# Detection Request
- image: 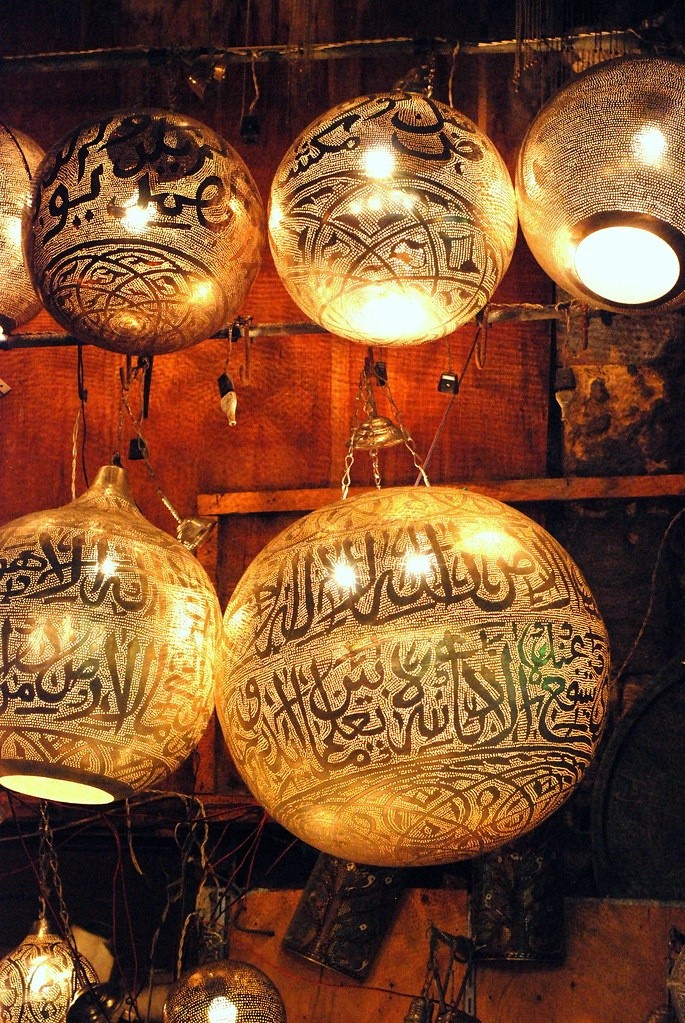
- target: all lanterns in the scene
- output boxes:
[0,920,289,1023]
[1,55,684,862]
